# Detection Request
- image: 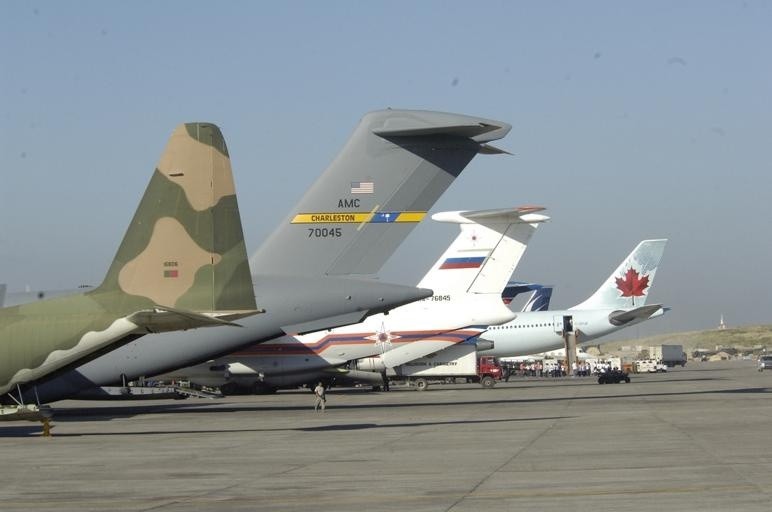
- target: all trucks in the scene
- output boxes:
[649,344,687,366]
[384,344,504,393]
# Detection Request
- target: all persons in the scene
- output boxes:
[372,369,382,392]
[381,367,391,392]
[313,380,328,414]
[508,360,612,378]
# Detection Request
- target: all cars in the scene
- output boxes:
[757,355,772,373]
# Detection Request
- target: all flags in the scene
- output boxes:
[437,249,492,271]
[350,181,375,194]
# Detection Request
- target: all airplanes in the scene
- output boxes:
[128,204,670,395]
[0,108,518,419]
